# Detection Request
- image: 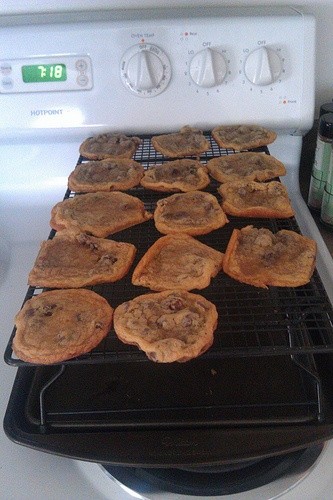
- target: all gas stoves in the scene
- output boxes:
[1,8,332,499]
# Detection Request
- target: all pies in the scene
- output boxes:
[221,224,318,290]
[153,191,229,236]
[140,159,211,192]
[28,232,136,287]
[151,124,211,157]
[211,124,277,151]
[68,157,144,191]
[217,180,295,218]
[206,152,287,183]
[10,289,114,366]
[79,132,144,158]
[131,233,225,291]
[114,289,218,363]
[49,191,154,239]
